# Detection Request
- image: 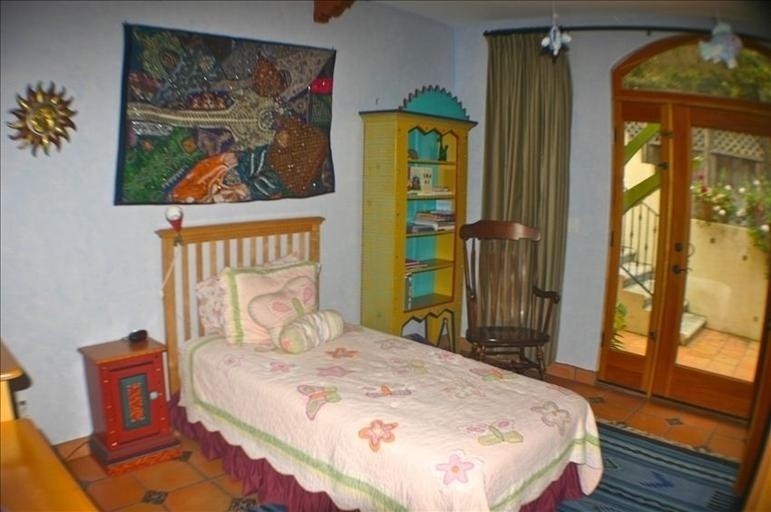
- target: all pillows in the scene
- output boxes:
[192,251,347,353]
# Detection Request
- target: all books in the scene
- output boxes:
[406,258,428,269]
[405,275,413,309]
[407,209,456,233]
[408,166,452,196]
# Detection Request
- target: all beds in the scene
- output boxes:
[160,213,591,511]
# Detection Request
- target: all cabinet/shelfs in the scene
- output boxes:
[358,108,479,357]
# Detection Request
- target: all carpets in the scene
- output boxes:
[552,413,744,512]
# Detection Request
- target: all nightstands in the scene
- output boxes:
[74,340,183,478]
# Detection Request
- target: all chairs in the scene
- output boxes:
[456,218,561,381]
